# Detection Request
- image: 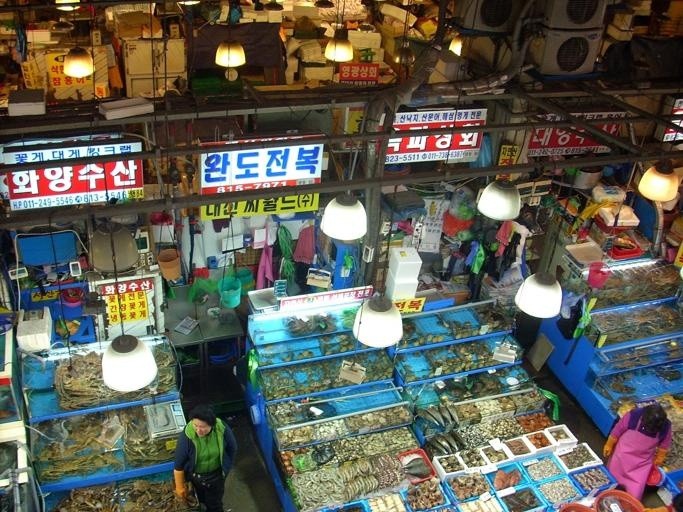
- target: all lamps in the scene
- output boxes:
[448,0,473,56]
[637,110,683,201]
[476,102,591,318]
[62,1,94,78]
[214,0,247,69]
[393,0,416,66]
[317,102,405,351]
[90,157,158,394]
[323,0,356,64]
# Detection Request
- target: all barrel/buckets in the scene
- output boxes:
[218,278,241,309]
[237,269,254,296]
[158,249,181,280]
[586,261,611,288]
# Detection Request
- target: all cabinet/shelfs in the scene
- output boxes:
[0,328,193,511]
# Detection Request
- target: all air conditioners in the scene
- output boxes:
[464,0,610,82]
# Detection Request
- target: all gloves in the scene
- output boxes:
[602,435,618,458]
[653,447,668,465]
[172,468,186,498]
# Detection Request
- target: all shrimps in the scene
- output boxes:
[290,453,406,512]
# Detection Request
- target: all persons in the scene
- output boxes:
[170,404,237,511]
[602,404,672,502]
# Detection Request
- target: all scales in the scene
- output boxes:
[143,400,186,439]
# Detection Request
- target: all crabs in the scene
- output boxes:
[37,346,198,512]
[586,303,683,370]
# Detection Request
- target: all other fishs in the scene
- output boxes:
[415,400,471,457]
[401,457,433,478]
